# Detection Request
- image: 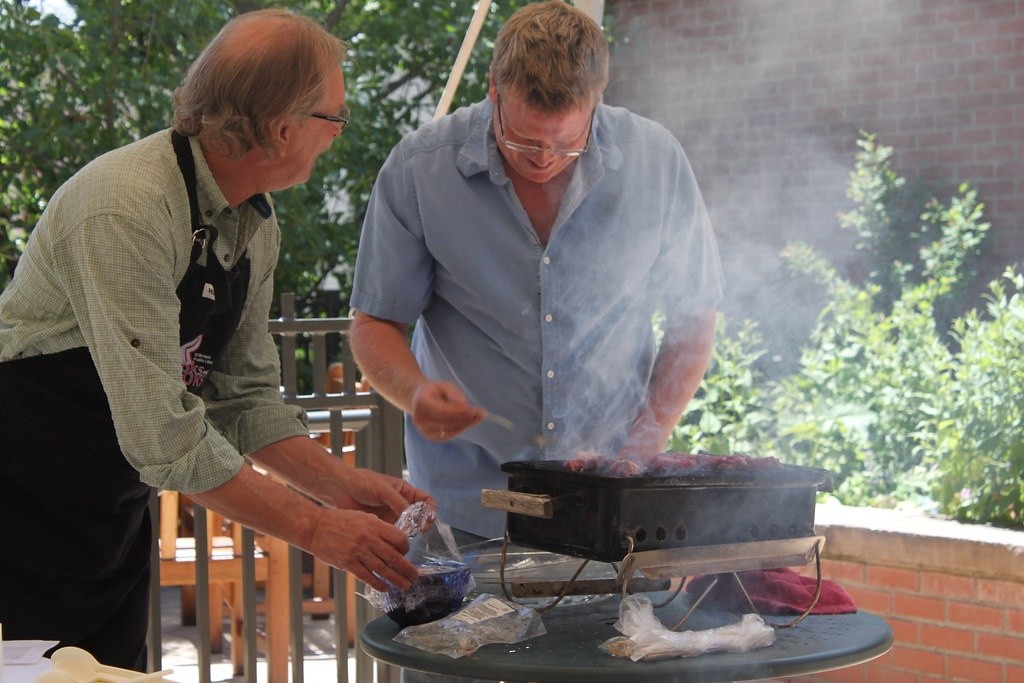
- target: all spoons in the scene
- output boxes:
[35,646,178,683]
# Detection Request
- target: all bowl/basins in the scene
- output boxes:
[377,561,471,629]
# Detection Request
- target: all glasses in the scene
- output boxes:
[497,92,596,156]
[311,105,350,129]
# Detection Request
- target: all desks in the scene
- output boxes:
[361,591,896,683]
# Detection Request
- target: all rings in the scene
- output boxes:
[438,425,445,437]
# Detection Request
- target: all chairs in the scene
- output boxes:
[160,484,290,683]
[178,363,371,655]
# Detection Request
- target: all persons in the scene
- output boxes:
[349,1,725,564]
[0,10,437,671]
